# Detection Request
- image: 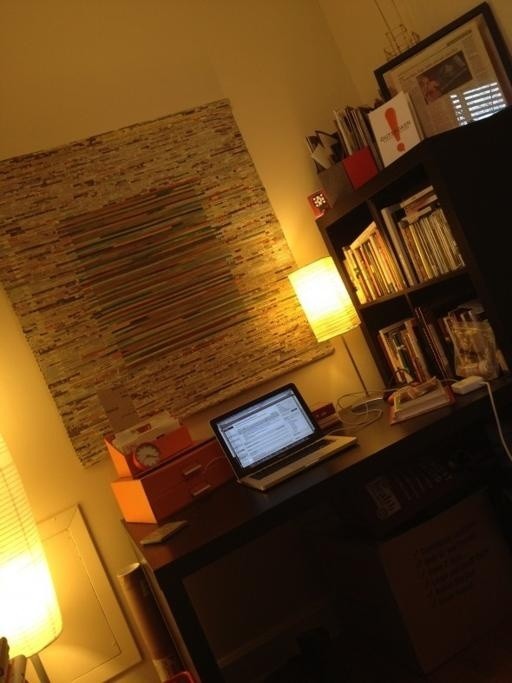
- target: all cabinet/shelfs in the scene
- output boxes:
[314,105,511,393]
[109,434,235,523]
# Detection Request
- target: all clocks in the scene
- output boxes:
[129,439,162,470]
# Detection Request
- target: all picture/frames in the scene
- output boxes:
[371,0,512,135]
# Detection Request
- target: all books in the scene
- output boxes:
[390,377,455,424]
[341,221,405,305]
[377,317,437,385]
[381,185,468,287]
[304,93,428,174]
[416,298,503,380]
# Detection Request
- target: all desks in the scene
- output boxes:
[117,378,511,683]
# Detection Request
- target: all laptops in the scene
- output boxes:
[209,382,358,491]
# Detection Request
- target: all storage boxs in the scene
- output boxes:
[340,145,381,187]
[377,493,511,683]
[316,161,355,208]
[425,608,509,683]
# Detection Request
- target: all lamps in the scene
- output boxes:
[287,255,384,409]
[1,434,67,682]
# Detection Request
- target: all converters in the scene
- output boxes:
[450,376,485,395]
